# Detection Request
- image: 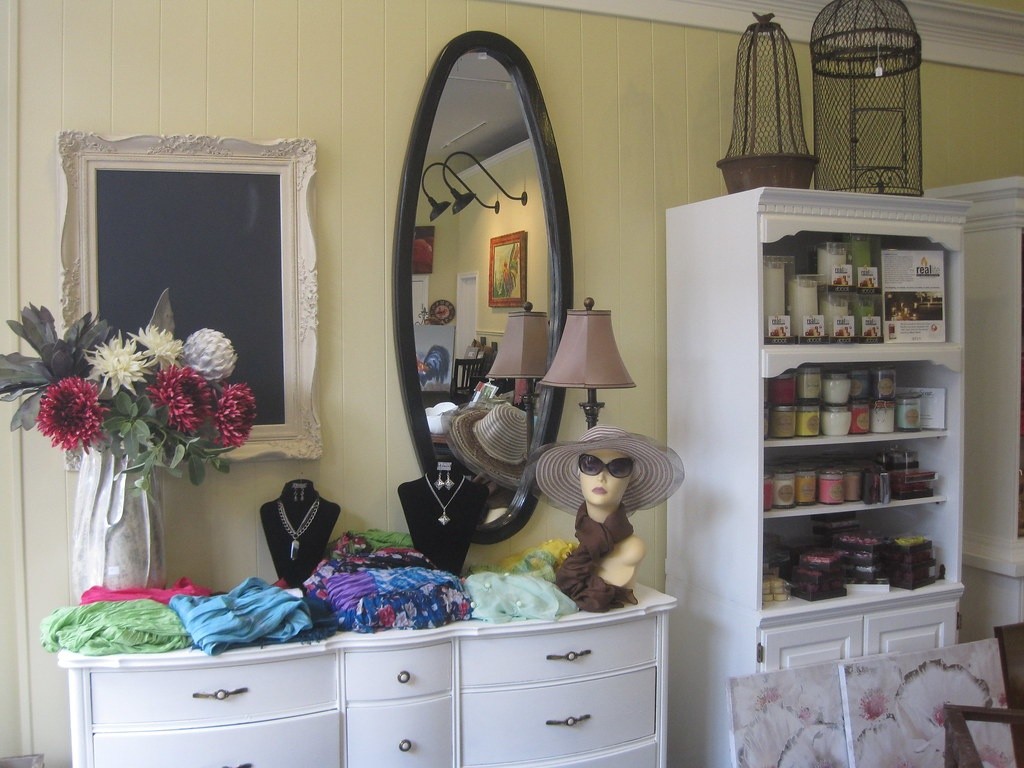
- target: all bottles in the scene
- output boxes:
[763,360,920,510]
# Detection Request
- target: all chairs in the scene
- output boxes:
[430,357,484,445]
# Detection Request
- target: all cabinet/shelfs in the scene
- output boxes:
[57,580,678,768]
[664,186,972,768]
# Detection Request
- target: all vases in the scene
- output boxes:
[72,447,169,602]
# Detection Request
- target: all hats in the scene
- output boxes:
[440,400,527,492]
[523,424,684,518]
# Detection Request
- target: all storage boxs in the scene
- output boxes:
[862,445,938,505]
[763,234,884,345]
[763,512,937,601]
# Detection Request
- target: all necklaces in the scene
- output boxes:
[425,473,466,525]
[277,494,319,562]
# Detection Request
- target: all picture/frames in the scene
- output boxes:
[52,128,327,465]
[488,230,528,308]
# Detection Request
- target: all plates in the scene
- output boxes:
[429,299,455,325]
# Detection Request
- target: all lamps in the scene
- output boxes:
[421,151,527,223]
[537,296,637,430]
[485,301,550,453]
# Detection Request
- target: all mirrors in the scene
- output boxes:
[391,29,578,548]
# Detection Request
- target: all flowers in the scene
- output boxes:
[0,300,258,507]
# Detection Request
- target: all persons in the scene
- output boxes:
[523,425,684,612]
[439,402,528,524]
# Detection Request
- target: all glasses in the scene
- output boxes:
[578,454,633,479]
